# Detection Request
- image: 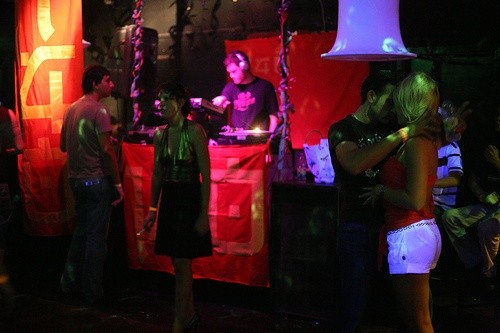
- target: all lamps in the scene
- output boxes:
[320,0,417,61]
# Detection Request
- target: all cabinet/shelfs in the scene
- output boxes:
[271,179,345,333]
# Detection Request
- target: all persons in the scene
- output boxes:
[60,65,125,311]
[190,51,278,143]
[134,80,212,333]
[326,72,442,333]
[431,105,500,292]
[358,72,443,333]
[0,100,30,222]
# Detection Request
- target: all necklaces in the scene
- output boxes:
[353,113,359,121]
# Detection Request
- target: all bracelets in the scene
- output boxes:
[148,206,157,212]
[113,183,122,188]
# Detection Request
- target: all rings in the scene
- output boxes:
[367,198,371,201]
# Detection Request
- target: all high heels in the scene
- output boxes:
[185,311,201,333]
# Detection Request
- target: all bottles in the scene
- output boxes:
[441,94,461,142]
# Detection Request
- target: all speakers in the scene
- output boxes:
[268,181,348,319]
[107,25,159,99]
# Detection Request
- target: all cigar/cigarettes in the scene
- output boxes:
[135,229,145,235]
